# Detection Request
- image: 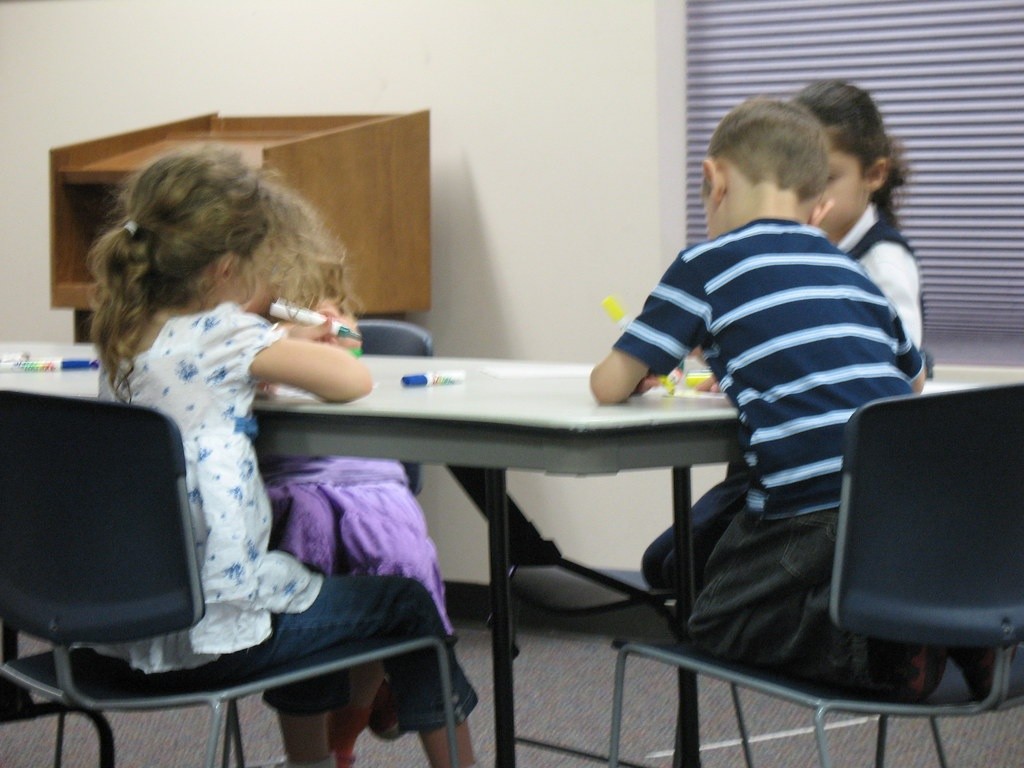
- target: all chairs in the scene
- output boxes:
[0,388,461,768]
[605,381,1024,768]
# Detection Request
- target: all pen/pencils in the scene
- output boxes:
[0,360,90,371]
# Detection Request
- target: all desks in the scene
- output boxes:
[0,344,739,768]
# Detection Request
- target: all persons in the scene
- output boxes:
[84,142,479,768]
[590,76,996,702]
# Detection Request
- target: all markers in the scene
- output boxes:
[601,296,675,394]
[401,375,459,388]
[269,298,369,344]
